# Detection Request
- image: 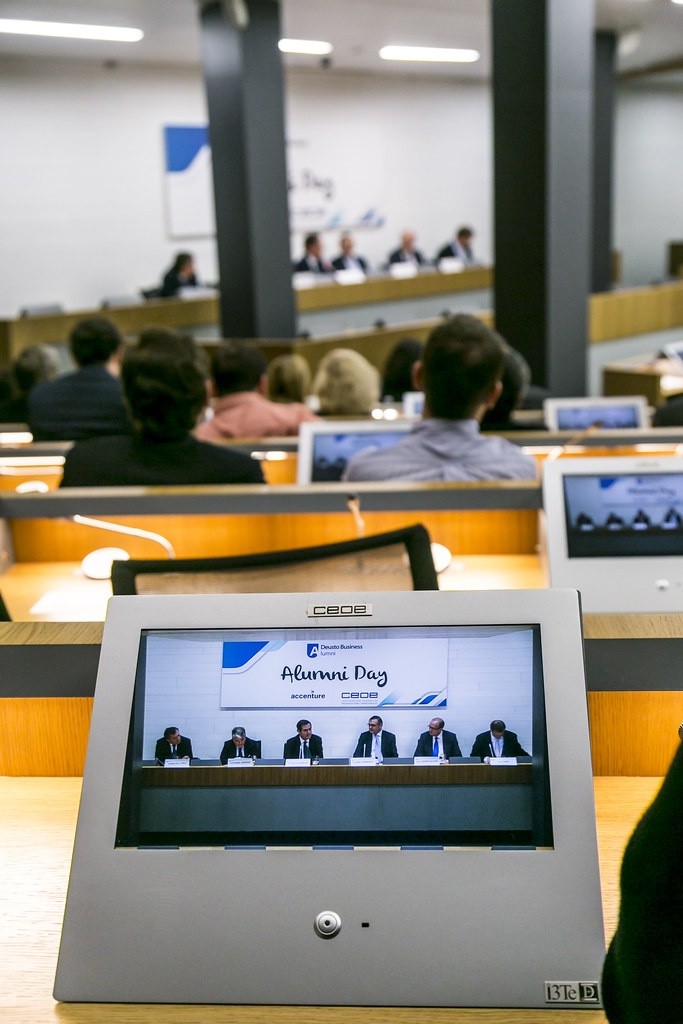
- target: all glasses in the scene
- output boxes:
[368,724,380,727]
[427,724,440,730]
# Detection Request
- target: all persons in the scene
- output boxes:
[577,508,681,526]
[160,255,195,298]
[601,719,683,1024]
[0,319,682,439]
[56,327,265,488]
[414,718,462,757]
[391,233,421,265]
[295,235,335,274]
[156,727,193,766]
[284,720,322,760]
[352,716,399,758]
[470,720,529,764]
[344,315,535,482]
[333,237,364,270]
[220,727,259,766]
[439,230,471,258]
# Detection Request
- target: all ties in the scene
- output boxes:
[171,744,177,755]
[374,734,379,756]
[495,739,500,758]
[304,741,307,758]
[433,736,439,756]
[238,747,242,757]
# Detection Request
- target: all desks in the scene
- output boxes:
[136,761,535,831]
[1,268,682,1023]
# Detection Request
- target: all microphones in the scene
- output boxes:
[488,744,494,757]
[363,744,366,757]
[299,746,302,760]
[72,516,175,560]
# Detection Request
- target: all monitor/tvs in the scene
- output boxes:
[298,423,421,490]
[50,595,606,1010]
[542,456,683,615]
[542,398,648,432]
